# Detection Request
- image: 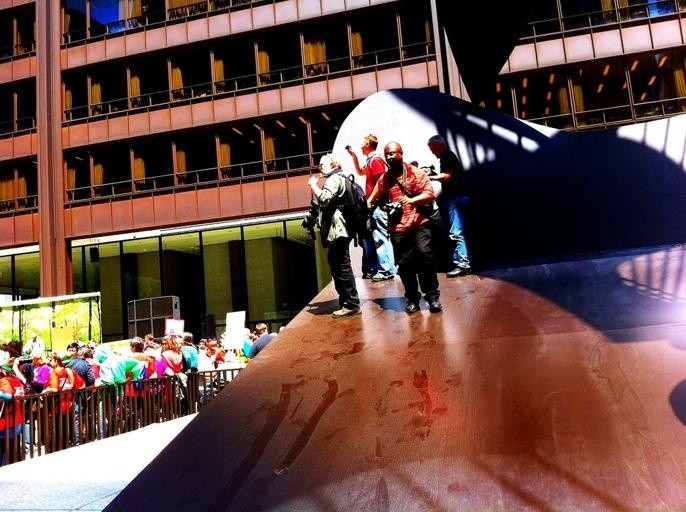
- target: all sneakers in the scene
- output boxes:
[332,308,361,318]
[362,270,394,282]
[406,302,419,313]
[431,300,441,312]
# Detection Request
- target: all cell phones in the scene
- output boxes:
[346,145,351,149]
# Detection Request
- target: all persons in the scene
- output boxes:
[426,135,473,278]
[377,141,441,312]
[306,153,360,317]
[0,323,284,466]
[348,134,395,282]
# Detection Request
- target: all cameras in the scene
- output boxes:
[386,201,403,218]
[303,201,319,232]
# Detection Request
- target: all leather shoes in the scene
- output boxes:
[447,266,471,277]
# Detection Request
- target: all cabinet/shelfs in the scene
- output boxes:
[127,295,180,340]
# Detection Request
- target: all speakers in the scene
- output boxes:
[200,313,215,339]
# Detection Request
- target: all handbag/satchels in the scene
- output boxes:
[334,174,367,233]
[429,210,443,228]
